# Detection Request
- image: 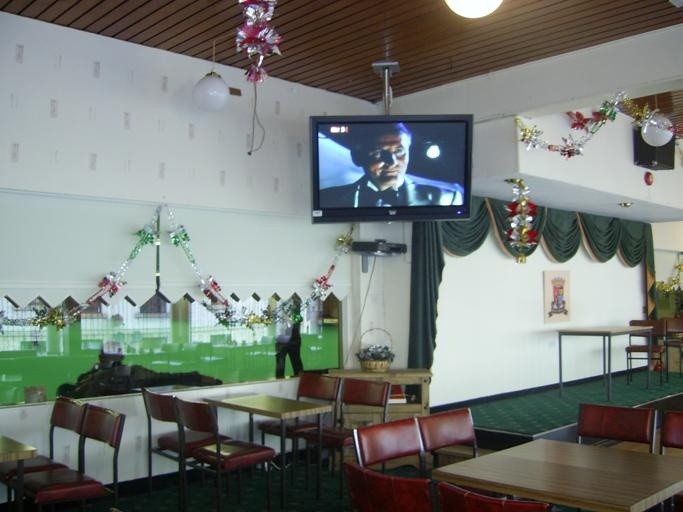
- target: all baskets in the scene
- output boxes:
[358,328,393,373]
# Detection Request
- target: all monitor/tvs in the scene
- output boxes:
[310,114,473,224]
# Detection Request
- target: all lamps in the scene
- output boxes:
[192,41,231,114]
[641,95,675,147]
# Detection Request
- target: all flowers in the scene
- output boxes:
[356,343,396,361]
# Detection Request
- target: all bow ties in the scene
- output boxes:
[361,186,398,207]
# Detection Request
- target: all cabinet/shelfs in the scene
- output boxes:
[328,366,435,429]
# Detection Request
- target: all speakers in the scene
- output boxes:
[635,125,675,172]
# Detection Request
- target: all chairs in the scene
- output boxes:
[659,317,683,373]
[626,319,668,387]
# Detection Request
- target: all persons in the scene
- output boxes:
[317,123,464,207]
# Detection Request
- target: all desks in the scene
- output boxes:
[558,326,654,402]
